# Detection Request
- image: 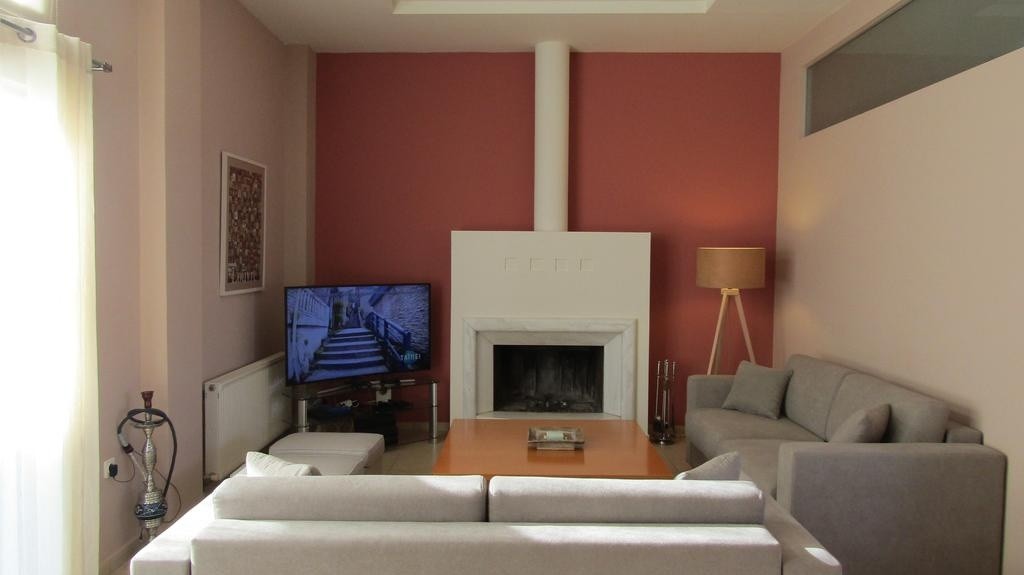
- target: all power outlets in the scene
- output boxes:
[104,457,114,479]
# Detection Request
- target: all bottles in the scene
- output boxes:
[652,361,678,446]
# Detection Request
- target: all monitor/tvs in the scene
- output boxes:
[284,282,431,386]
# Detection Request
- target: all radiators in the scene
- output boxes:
[205,351,292,480]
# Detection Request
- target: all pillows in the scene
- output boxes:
[832,404,890,443]
[674,452,740,480]
[246,451,323,476]
[722,360,793,420]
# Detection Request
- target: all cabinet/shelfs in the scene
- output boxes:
[282,375,441,445]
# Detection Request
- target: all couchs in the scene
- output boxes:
[130,468,840,575]
[685,353,1007,575]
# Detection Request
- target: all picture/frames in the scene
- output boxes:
[220,151,266,297]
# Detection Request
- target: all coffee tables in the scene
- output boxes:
[433,421,673,480]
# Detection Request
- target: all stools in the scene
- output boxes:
[269,432,385,475]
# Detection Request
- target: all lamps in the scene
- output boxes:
[696,247,765,375]
